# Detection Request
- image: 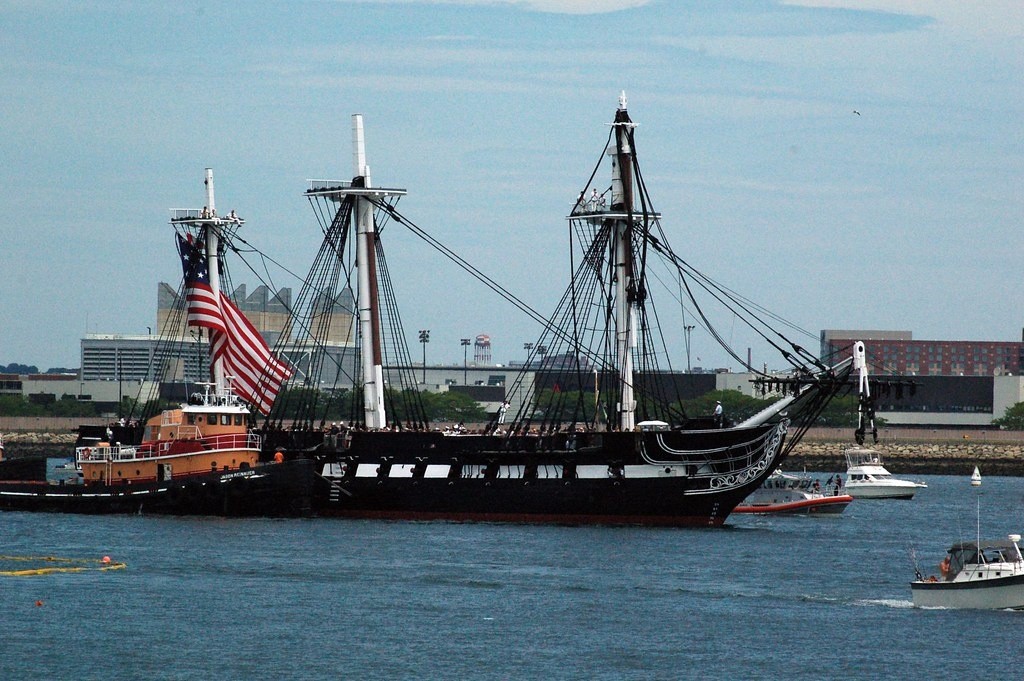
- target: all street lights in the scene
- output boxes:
[683,324,695,372]
[523,341,532,367]
[418,329,432,385]
[461,338,471,386]
[537,345,548,363]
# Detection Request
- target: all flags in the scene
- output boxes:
[178,234,295,418]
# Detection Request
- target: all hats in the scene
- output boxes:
[339,421,343,424]
[716,401,721,404]
[332,422,336,425]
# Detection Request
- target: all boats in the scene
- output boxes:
[906,492,1024,612]
[75,166,263,491]
[243,89,878,532]
[844,445,928,500]
[730,467,854,515]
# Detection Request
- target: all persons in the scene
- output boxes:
[941,556,950,582]
[713,400,722,429]
[813,478,821,495]
[210,390,243,407]
[110,435,117,460]
[274,446,286,464]
[121,414,126,427]
[327,421,664,436]
[832,474,842,496]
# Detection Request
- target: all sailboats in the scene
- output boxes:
[969,465,983,488]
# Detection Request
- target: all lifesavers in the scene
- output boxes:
[82,447,92,460]
